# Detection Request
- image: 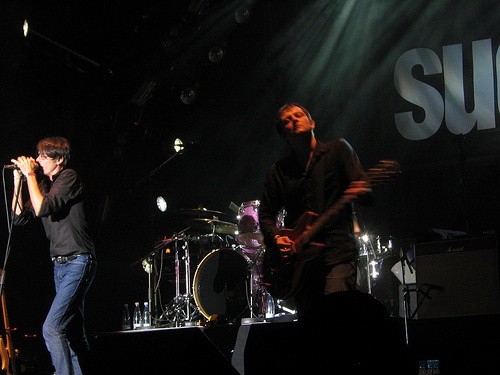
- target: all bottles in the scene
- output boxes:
[133,302,142,330]
[426,360,440,375]
[417,361,426,375]
[121,303,132,330]
[142,302,149,328]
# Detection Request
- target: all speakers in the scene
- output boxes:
[397,234,500,321]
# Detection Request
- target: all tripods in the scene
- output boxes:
[152,235,202,328]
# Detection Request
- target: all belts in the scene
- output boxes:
[52,253,83,264]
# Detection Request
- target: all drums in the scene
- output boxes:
[237,200,269,245]
[190,244,276,322]
[355,231,396,263]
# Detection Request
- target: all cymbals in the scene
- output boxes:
[180,207,227,216]
[194,217,236,234]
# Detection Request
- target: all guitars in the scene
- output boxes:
[273,161,401,265]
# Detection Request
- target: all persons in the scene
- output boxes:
[257,102,373,321]
[9,136,98,375]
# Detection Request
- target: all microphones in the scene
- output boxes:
[4,162,38,170]
[422,293,432,300]
[429,284,443,291]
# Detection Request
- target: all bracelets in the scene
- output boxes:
[25,172,36,178]
[274,236,281,243]
